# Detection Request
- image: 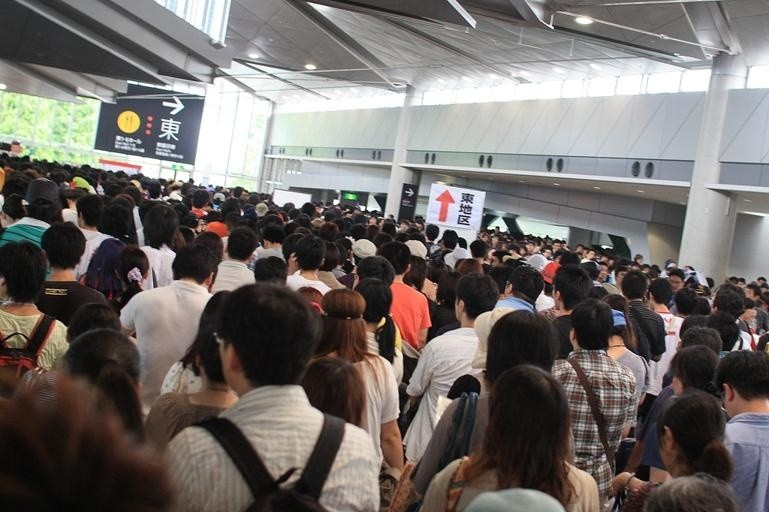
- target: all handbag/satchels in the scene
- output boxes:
[378,461,400,511]
[390,393,477,512]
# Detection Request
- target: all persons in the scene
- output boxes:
[253,256,286,284]
[497,268,541,314]
[258,236,286,256]
[432,221,539,269]
[194,231,223,263]
[78,238,124,302]
[286,236,332,297]
[702,311,741,352]
[404,271,500,467]
[120,242,222,419]
[666,257,697,277]
[333,236,350,280]
[539,233,667,265]
[754,276,769,350]
[146,337,241,449]
[350,236,376,255]
[612,265,666,275]
[66,301,124,340]
[612,391,732,512]
[686,278,709,311]
[376,236,432,243]
[612,277,686,482]
[709,287,754,352]
[61,327,159,457]
[408,243,432,271]
[354,278,403,386]
[210,223,258,292]
[110,223,194,242]
[0,240,70,373]
[539,265,592,321]
[443,307,522,402]
[623,325,724,475]
[420,362,599,512]
[305,289,403,471]
[162,280,383,512]
[410,306,575,512]
[669,345,729,421]
[35,222,109,325]
[258,192,432,236]
[622,273,666,363]
[294,284,324,312]
[0,139,258,223]
[375,242,432,350]
[640,473,740,512]
[552,301,634,512]
[713,350,769,512]
[592,265,612,301]
[302,358,420,512]
[158,286,238,399]
[110,245,149,317]
[350,255,396,291]
[0,372,173,511]
[709,276,754,287]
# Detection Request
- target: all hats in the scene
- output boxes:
[254,203,268,217]
[539,262,560,284]
[23,178,59,210]
[519,254,547,273]
[213,193,226,201]
[352,238,378,258]
[405,240,427,259]
[444,248,472,271]
[503,254,522,263]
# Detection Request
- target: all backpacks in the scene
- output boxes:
[1,312,54,378]
[191,412,345,512]
